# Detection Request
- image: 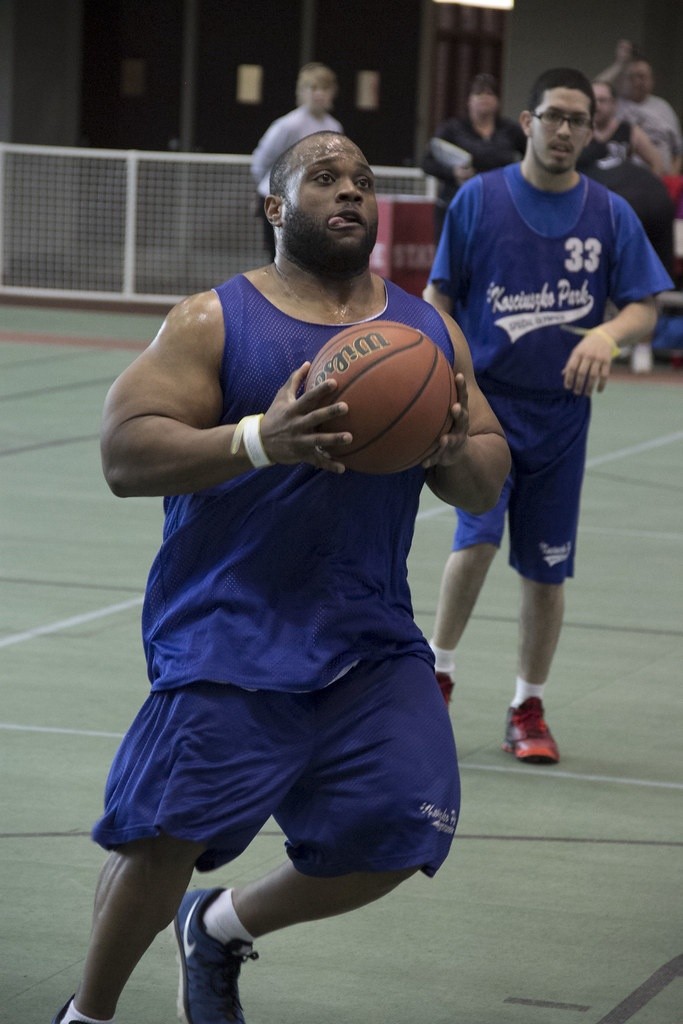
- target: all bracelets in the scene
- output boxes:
[230,413,278,470]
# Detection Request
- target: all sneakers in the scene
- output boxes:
[502,697,559,762]
[169,886,253,1024]
[49,993,88,1024]
[435,672,455,707]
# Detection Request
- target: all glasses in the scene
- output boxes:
[532,112,593,132]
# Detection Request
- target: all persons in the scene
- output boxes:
[583,36,682,379]
[427,67,675,766]
[248,61,345,268]
[54,132,512,1024]
[419,73,527,259]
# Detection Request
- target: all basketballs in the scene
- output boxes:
[302,319,457,475]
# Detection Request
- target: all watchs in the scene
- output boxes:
[577,327,624,361]
